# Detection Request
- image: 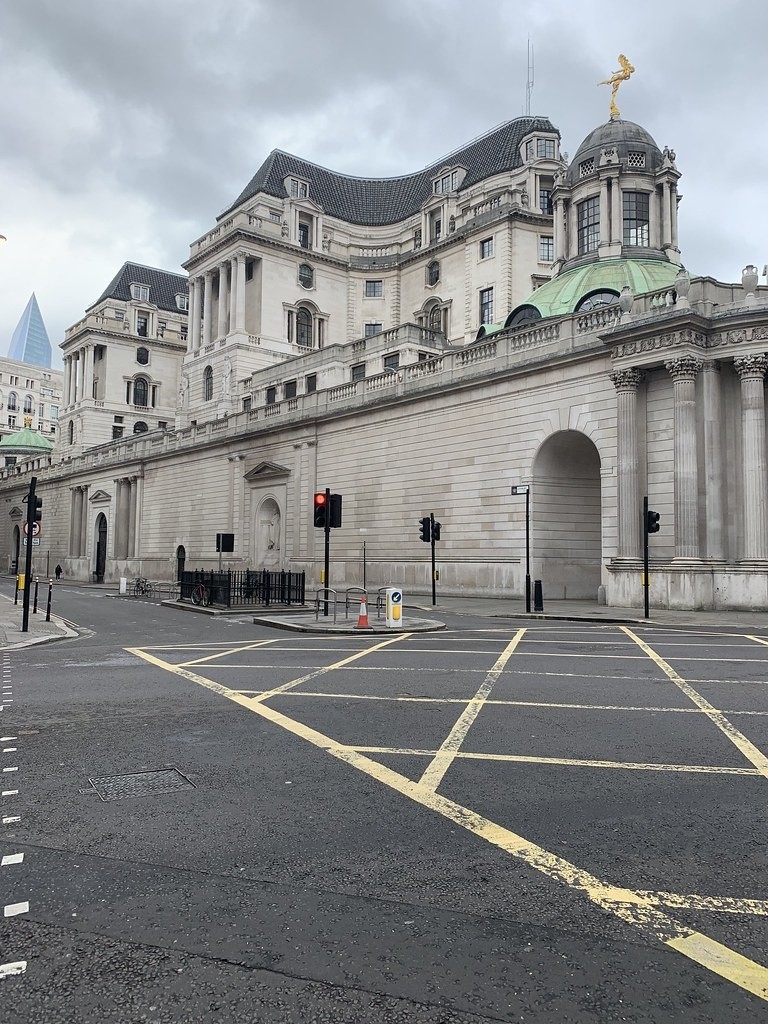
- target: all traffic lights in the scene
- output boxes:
[648,511,661,533]
[28,495,42,523]
[419,516,430,542]
[314,493,326,527]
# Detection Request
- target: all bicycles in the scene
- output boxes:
[133,577,153,598]
[191,577,212,607]
[240,576,267,600]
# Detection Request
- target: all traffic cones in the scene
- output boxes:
[352,596,373,629]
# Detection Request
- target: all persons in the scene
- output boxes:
[220,355,233,395]
[176,372,189,409]
[597,54,635,114]
[55,564,62,582]
[268,507,280,551]
